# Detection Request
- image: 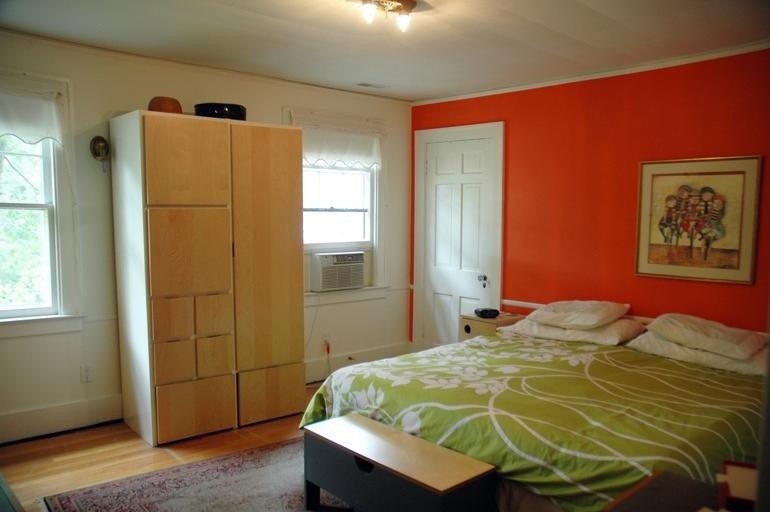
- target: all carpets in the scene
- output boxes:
[43,436,354,512]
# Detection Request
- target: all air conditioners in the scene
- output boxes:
[311,250,365,292]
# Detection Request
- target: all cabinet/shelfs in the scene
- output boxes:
[458,310,526,343]
[107,109,308,448]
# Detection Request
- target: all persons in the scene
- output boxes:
[658,185,726,243]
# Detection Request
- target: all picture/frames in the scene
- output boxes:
[633,155,762,286]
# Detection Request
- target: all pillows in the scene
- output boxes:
[496,318,646,346]
[625,331,770,380]
[642,312,770,362]
[526,300,631,330]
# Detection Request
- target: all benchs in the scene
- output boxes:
[304,413,500,512]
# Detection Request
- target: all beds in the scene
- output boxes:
[298,333,770,512]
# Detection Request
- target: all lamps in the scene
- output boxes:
[352,0,418,34]
